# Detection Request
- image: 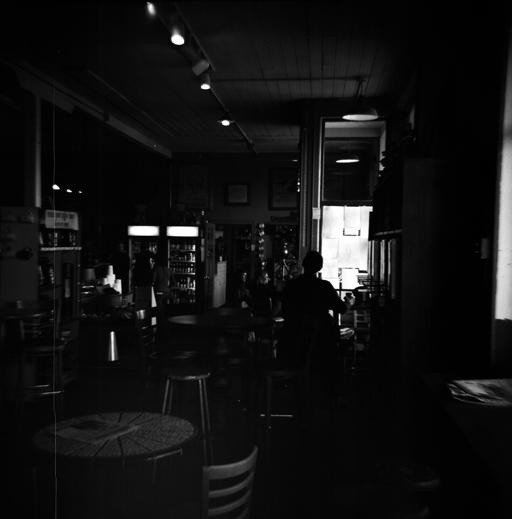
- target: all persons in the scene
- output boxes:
[278,250,345,357]
[152,256,174,298]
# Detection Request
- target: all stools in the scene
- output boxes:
[256,370,309,449]
[161,370,213,465]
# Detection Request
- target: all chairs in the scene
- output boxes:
[16,297,69,419]
[170,446,259,518]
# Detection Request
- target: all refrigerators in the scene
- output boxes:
[127,224,199,326]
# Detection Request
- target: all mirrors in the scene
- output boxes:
[321,136,380,206]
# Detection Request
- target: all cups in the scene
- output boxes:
[100,265,123,294]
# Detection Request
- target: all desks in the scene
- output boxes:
[32,411,200,519]
[165,308,252,390]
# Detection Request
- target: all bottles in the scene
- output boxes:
[169,243,196,303]
[131,241,156,265]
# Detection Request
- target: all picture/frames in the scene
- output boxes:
[219,182,252,203]
[266,168,299,210]
[174,163,215,212]
